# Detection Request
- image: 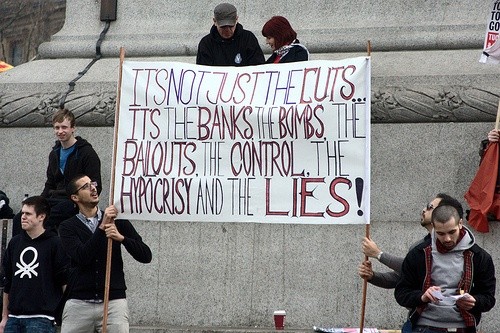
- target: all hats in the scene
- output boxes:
[213,3,237,26]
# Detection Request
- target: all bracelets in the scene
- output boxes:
[377,252,383,260]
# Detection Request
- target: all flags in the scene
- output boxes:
[464,102,500,233]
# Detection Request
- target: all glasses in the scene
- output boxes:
[427,204,435,210]
[73,182,98,195]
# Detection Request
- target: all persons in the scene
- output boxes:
[38,109,103,222]
[262,16,309,64]
[196,3,265,66]
[0,190,71,333]
[59,175,152,333]
[479,129,499,166]
[359,193,497,333]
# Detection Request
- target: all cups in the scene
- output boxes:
[274,310,286,330]
